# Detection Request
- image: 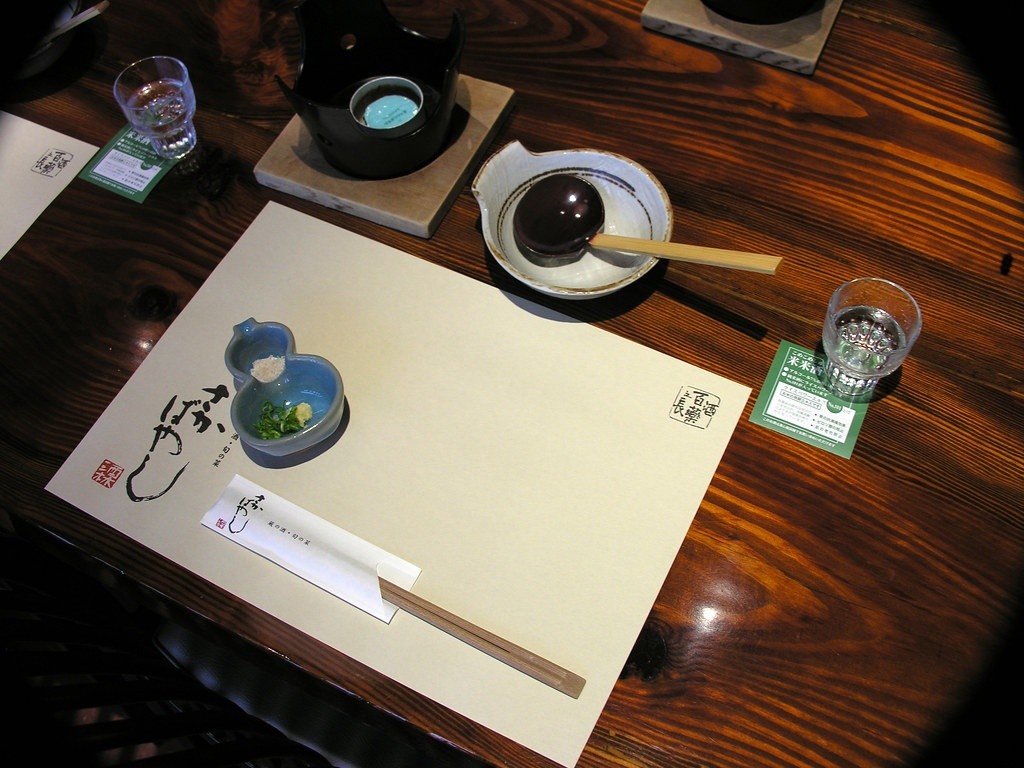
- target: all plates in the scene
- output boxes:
[0,0,107,104]
[225,317,345,457]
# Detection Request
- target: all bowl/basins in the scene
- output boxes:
[0,0,81,82]
[471,138,674,299]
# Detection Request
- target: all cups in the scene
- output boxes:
[822,277,922,396]
[113,56,196,160]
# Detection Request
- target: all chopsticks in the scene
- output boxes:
[377,574,586,699]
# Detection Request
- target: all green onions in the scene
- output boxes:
[252,400,307,440]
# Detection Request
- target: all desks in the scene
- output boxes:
[0,0,1024,768]
